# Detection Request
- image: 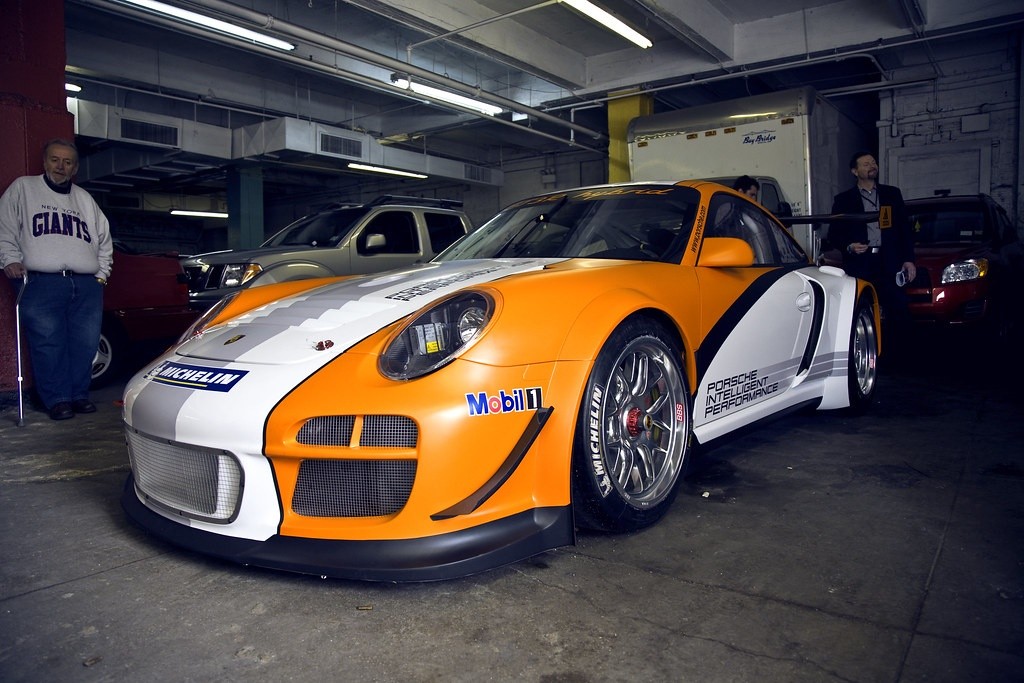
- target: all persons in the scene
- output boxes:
[0,139,114,420]
[714,175,761,238]
[824,149,916,413]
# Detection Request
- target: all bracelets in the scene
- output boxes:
[848,246,852,254]
[96,279,107,286]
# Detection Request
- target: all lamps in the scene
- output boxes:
[555,0,654,50]
[169,209,229,218]
[390,72,511,116]
[113,0,298,51]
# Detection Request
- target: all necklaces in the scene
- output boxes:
[860,188,878,211]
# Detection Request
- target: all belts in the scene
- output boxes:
[866,246,881,254]
[58,270,72,276]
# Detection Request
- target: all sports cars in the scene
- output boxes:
[120,176,885,585]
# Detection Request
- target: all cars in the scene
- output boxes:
[89,243,196,391]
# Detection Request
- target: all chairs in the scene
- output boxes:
[647,228,676,252]
[738,214,776,264]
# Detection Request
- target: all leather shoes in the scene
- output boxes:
[73,398,97,413]
[49,405,76,420]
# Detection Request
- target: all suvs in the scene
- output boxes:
[875,191,1024,356]
[176,193,475,313]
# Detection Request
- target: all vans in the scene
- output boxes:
[708,175,795,243]
